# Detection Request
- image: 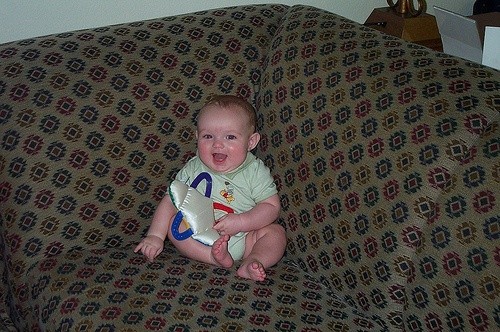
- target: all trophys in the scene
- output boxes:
[363,0,444,54]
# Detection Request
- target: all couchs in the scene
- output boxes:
[0,0,500,332]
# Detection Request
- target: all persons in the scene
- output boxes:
[133,95,289,282]
